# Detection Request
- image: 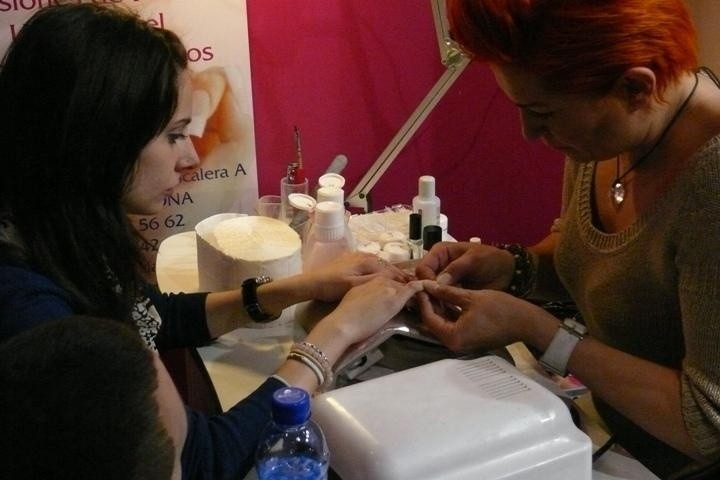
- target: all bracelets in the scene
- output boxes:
[286,340,336,386]
[497,240,536,298]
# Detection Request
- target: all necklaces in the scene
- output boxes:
[608,66,700,207]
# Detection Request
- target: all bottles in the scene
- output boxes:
[254,388,329,480]
[300,174,353,267]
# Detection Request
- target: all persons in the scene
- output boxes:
[402,0,720,480]
[0,314,190,480]
[1,1,427,480]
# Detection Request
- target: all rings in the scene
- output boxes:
[376,256,385,264]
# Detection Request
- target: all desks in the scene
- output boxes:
[154,205,666,480]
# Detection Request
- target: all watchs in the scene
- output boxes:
[535,315,590,379]
[240,272,286,323]
